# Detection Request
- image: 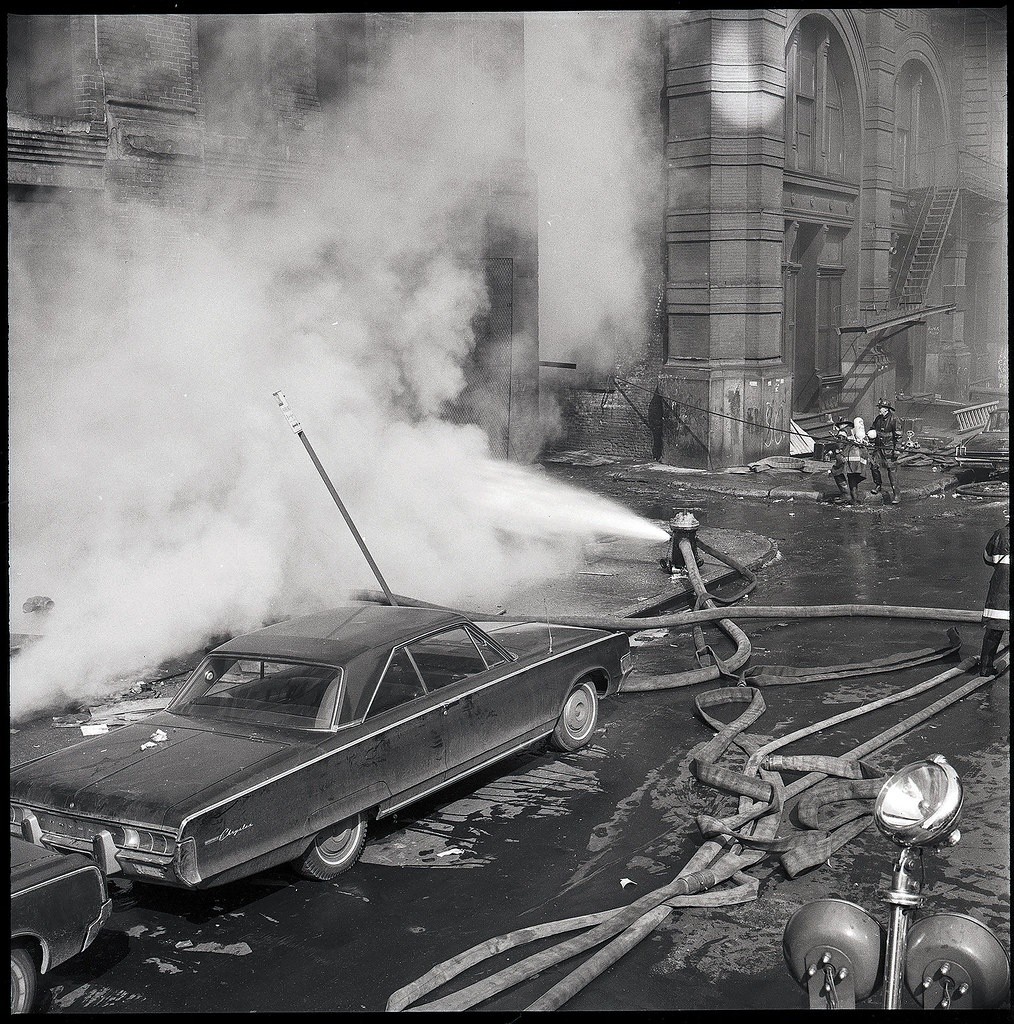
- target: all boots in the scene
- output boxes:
[835,484,852,502]
[871,475,882,494]
[891,488,901,503]
[848,483,865,503]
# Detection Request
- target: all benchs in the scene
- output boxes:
[287,676,431,715]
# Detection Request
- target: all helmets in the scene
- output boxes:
[835,417,855,428]
[874,398,895,412]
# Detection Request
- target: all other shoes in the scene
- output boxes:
[980,664,998,676]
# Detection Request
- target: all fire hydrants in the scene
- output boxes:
[669,510,707,577]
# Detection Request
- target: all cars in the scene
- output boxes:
[11,606,635,891]
[10,835,115,1015]
[954,406,1009,472]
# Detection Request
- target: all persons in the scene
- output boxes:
[980,520,1010,677]
[830,417,870,505]
[863,397,903,504]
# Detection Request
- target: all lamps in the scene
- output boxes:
[782,754,1010,1011]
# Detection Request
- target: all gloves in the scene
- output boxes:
[891,451,900,462]
[867,429,877,439]
